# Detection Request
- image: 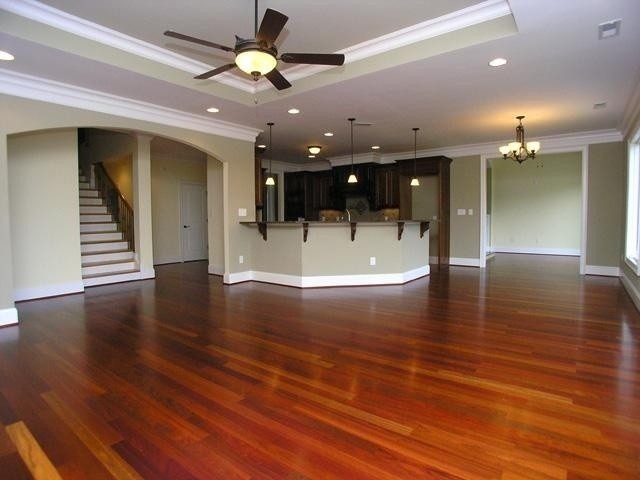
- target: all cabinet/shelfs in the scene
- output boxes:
[283,155,453,272]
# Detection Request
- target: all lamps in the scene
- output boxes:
[235,48,277,78]
[307,146,321,154]
[408,127,421,186]
[499,115,542,163]
[346,117,359,183]
[265,122,276,185]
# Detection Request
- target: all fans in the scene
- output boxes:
[163,1,344,93]
